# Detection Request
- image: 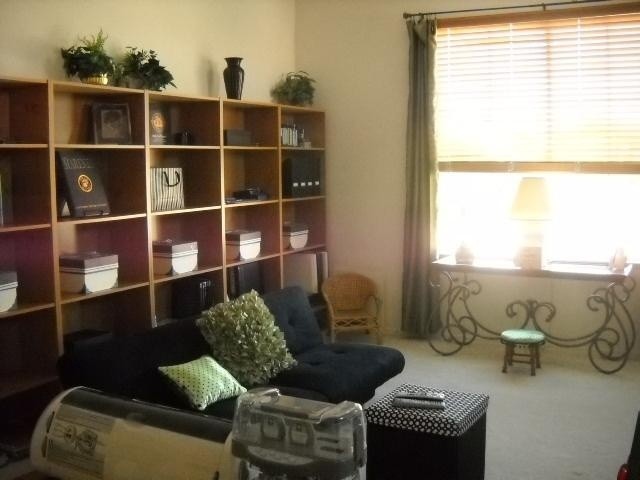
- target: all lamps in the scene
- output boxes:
[509,175,554,273]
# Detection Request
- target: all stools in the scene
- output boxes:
[501,328,547,377]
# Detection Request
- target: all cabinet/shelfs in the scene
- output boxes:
[0,76,328,463]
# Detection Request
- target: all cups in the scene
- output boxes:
[176,133,192,145]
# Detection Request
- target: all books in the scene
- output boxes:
[280,123,298,148]
[55,150,111,219]
[168,273,216,320]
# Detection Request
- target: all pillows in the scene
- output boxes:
[156,353,248,411]
[195,289,300,389]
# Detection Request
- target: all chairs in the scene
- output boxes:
[319,272,386,345]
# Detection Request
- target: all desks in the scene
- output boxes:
[363,382,490,480]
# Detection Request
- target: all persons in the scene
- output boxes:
[102,110,122,129]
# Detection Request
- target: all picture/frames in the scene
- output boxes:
[90,98,132,146]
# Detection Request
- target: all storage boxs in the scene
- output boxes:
[223,127,255,145]
[279,153,322,198]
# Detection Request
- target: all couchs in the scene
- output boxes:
[58,285,405,436]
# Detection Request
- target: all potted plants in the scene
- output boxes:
[59,30,118,85]
[118,42,179,91]
